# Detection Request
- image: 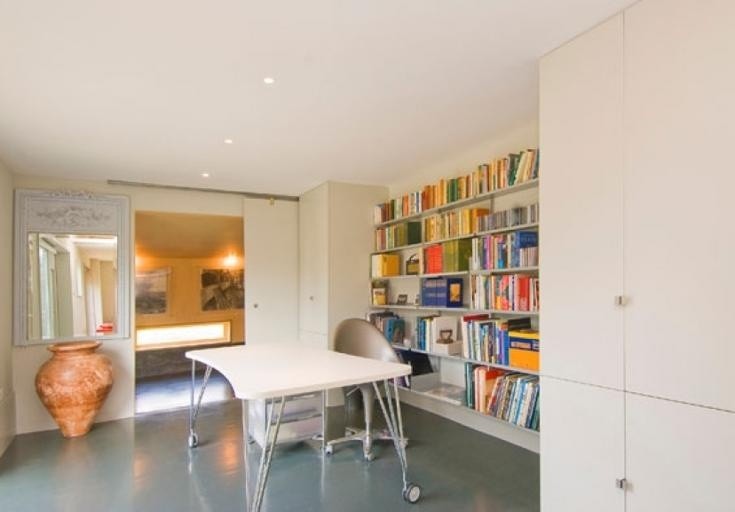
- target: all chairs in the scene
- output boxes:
[325,319,411,460]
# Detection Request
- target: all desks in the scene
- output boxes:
[183,343,422,512]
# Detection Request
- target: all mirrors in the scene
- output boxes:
[13,188,132,346]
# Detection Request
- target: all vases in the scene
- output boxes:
[35,341,114,439]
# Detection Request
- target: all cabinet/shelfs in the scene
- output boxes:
[368,181,539,453]
[539,375,735,512]
[247,387,327,450]
[538,1,735,412]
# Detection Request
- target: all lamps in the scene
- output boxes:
[136,320,231,350]
